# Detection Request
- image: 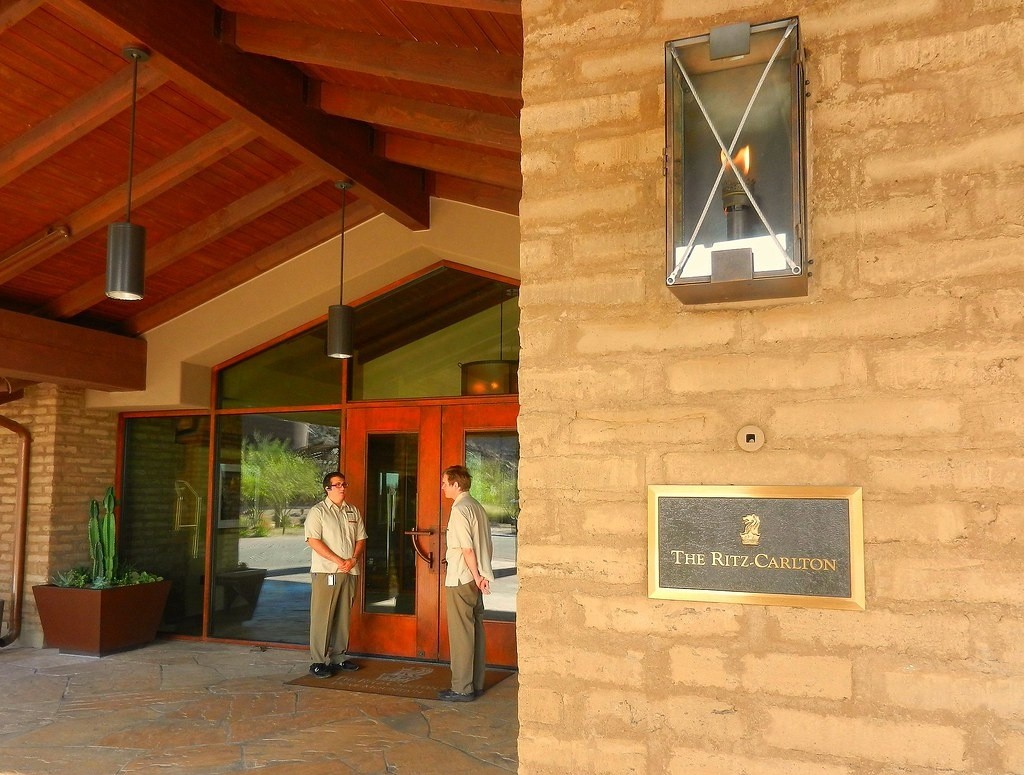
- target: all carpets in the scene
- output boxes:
[284,658,515,702]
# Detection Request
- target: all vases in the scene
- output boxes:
[201,568,266,622]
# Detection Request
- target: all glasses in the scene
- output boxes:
[332,482,349,488]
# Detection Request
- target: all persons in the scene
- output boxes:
[303,471,368,680]
[438,465,497,702]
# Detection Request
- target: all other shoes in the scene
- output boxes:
[474,688,485,697]
[438,689,474,702]
[310,663,332,679]
[329,660,358,672]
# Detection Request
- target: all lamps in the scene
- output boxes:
[458,288,518,397]
[105,43,150,301]
[328,179,354,358]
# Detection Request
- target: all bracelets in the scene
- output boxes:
[352,555,359,562]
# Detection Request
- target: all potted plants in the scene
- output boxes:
[31,486,174,657]
